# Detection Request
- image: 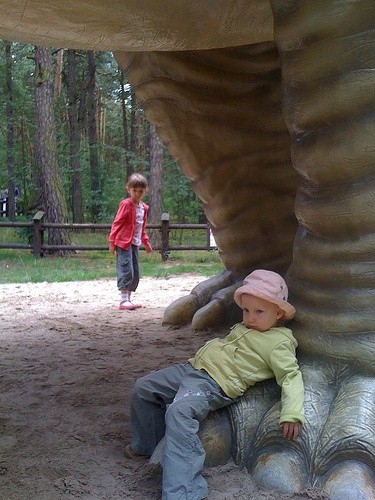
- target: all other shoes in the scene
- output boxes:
[120,300,136,309]
[134,303,142,308]
[126,445,139,458]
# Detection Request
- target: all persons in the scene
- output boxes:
[126,268,305,500]
[108,172,152,310]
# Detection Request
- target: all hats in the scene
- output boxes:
[234,269,296,320]
